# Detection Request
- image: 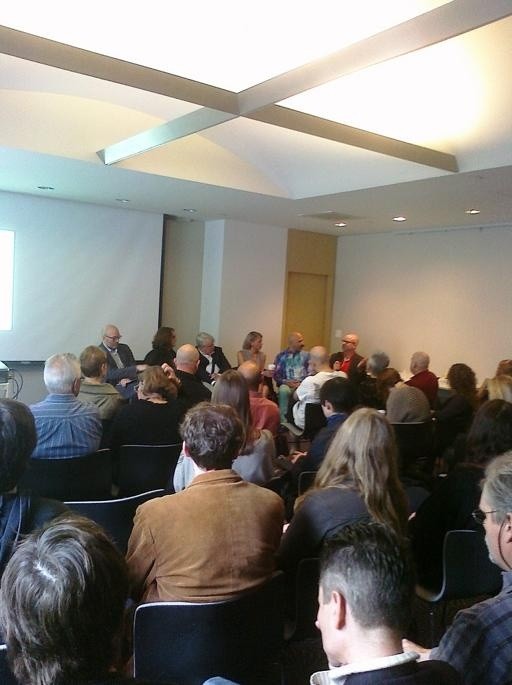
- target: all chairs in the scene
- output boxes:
[280,403,327,451]
[287,558,317,641]
[414,529,502,649]
[297,471,317,495]
[17,448,112,503]
[63,488,166,558]
[259,476,280,498]
[389,417,436,478]
[132,568,284,684]
[112,444,183,497]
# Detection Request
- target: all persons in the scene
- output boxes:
[0,325,511,684]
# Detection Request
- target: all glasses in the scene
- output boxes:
[473,508,499,522]
[107,335,120,340]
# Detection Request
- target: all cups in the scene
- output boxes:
[378,410,385,416]
[268,364,276,374]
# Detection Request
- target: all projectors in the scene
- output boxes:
[0,362,10,383]
